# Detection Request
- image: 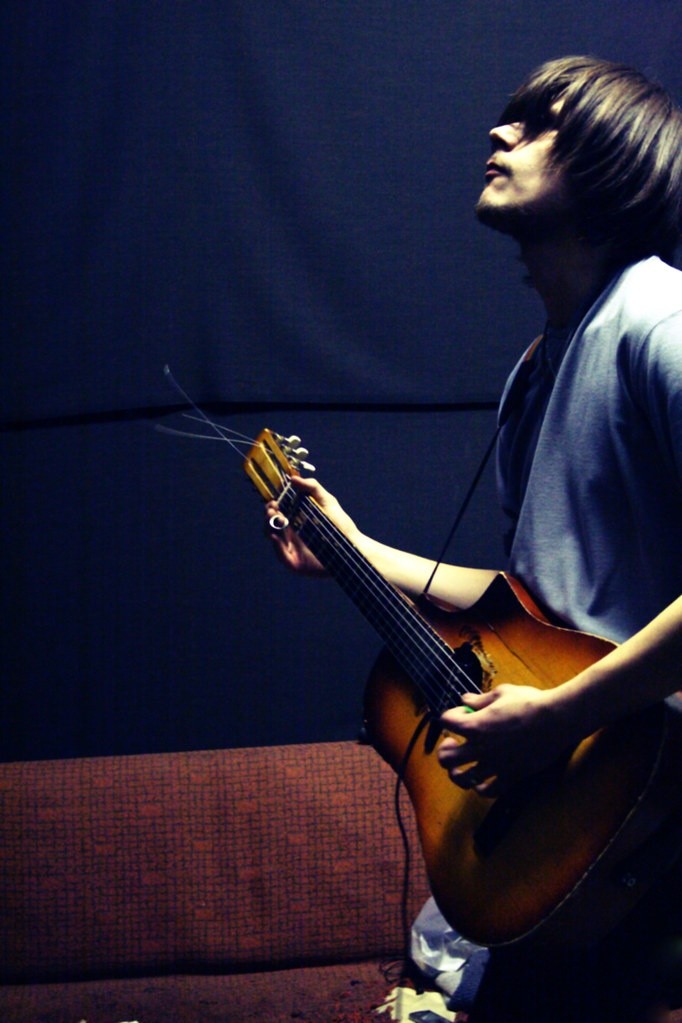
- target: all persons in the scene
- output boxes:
[272,51,681,1022]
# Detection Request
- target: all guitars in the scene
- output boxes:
[242,426,674,955]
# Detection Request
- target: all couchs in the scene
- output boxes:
[0,728,682,1023]
